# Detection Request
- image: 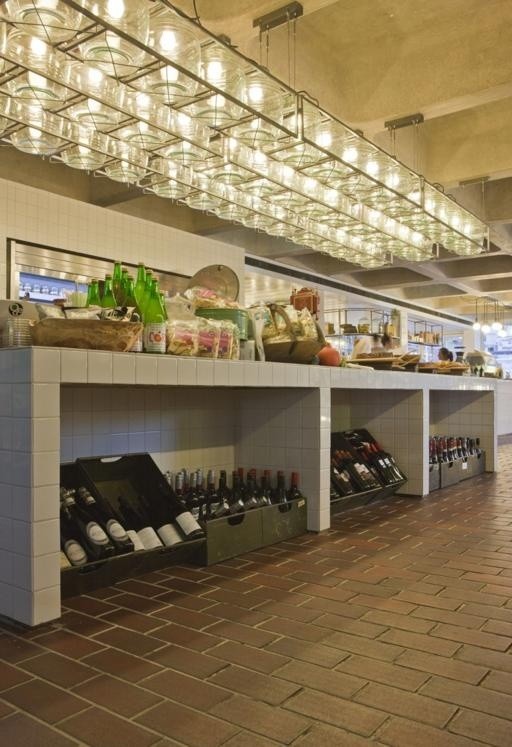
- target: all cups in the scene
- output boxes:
[357,324,371,333]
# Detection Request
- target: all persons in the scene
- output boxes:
[437,347,453,362]
[381,334,393,352]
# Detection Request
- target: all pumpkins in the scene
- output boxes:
[317,342,340,367]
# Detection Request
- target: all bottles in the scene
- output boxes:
[87,259,167,353]
[330,440,404,500]
[474,362,484,377]
[60,524,87,565]
[420,331,439,344]
[79,483,135,554]
[430,434,482,465]
[105,467,302,552]
[378,319,396,337]
[60,487,115,558]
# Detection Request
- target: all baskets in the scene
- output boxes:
[264,303,326,363]
